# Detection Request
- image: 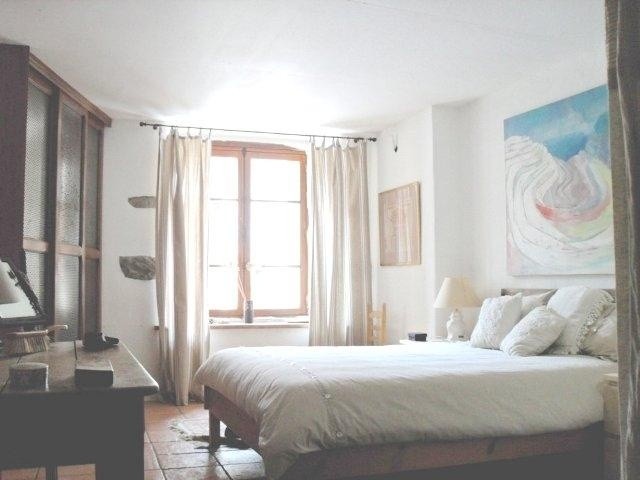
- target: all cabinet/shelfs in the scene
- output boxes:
[0,41,114,341]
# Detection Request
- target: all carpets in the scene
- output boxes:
[167,411,228,450]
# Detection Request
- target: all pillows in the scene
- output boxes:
[471,288,620,356]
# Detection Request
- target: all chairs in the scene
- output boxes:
[366,301,386,346]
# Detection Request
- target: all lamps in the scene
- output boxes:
[433,275,488,339]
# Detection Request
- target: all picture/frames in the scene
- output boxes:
[377,180,424,267]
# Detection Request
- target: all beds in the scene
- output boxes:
[195,288,620,480]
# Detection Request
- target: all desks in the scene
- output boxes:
[0,334,161,480]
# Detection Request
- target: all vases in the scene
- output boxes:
[244,300,253,323]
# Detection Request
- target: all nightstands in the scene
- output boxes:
[401,339,471,344]
[603,373,620,480]
